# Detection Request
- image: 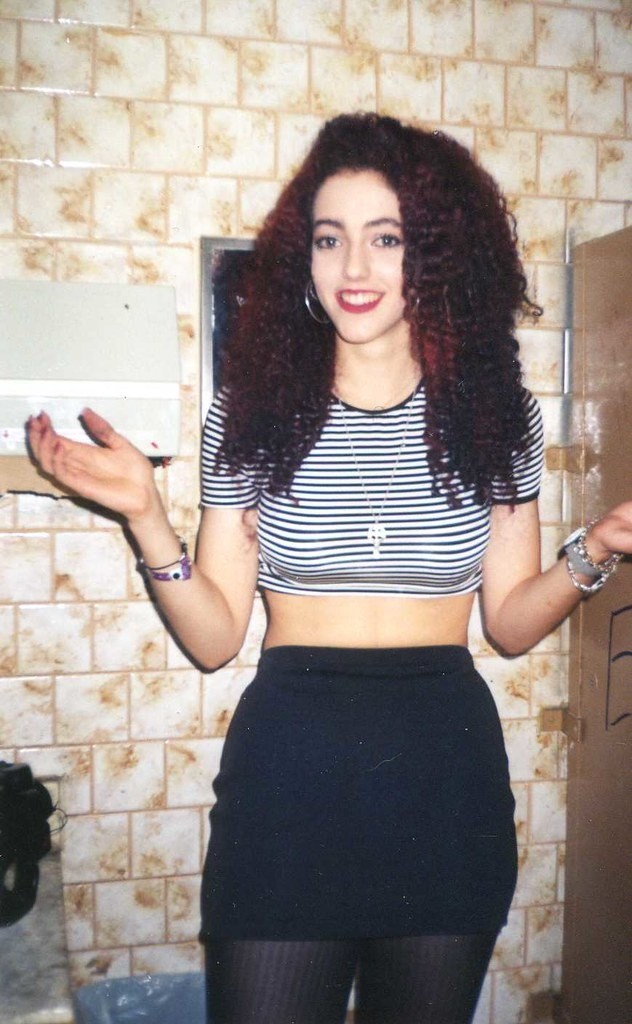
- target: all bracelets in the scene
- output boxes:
[136,534,192,582]
[561,519,623,594]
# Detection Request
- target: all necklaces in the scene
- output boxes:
[333,380,417,555]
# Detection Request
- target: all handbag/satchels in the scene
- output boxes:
[0,760,55,928]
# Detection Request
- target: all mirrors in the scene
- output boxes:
[199,236,263,426]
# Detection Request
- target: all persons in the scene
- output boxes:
[22,113,631,1024]
